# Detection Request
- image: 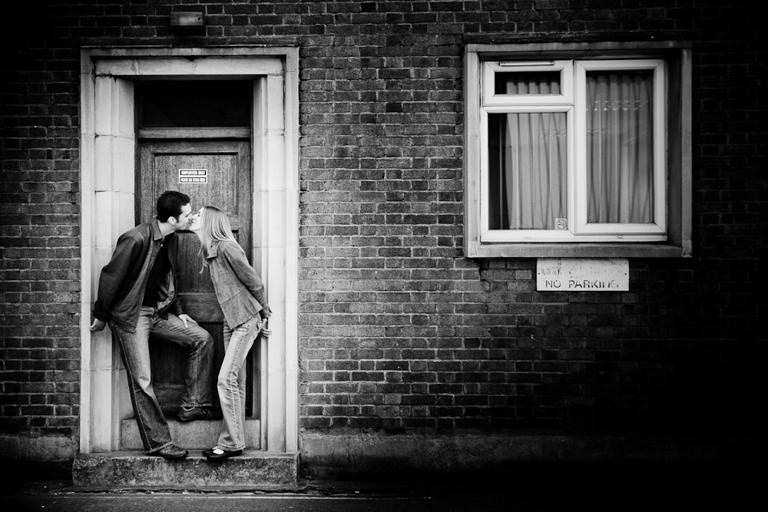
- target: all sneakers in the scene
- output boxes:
[177,405,224,423]
[142,443,189,460]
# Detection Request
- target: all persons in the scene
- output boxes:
[189,205,272,462]
[91,191,215,462]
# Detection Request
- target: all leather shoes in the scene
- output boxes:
[201,446,242,461]
